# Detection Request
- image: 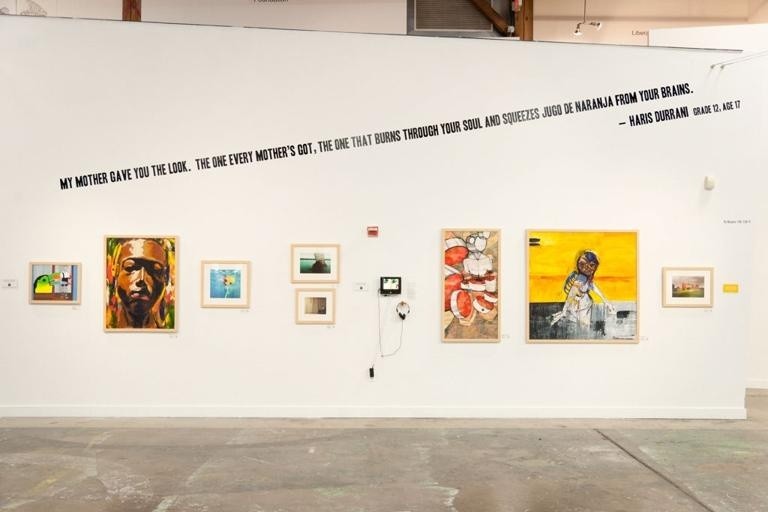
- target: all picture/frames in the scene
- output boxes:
[295,287,335,325]
[28,260,82,304]
[662,266,714,309]
[441,228,501,343]
[525,228,640,345]
[290,243,340,284]
[200,260,251,308]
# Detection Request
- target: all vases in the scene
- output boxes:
[104,234,179,334]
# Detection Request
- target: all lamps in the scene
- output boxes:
[574,0,601,35]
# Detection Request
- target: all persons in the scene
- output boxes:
[311,255,327,273]
[105,239,169,329]
